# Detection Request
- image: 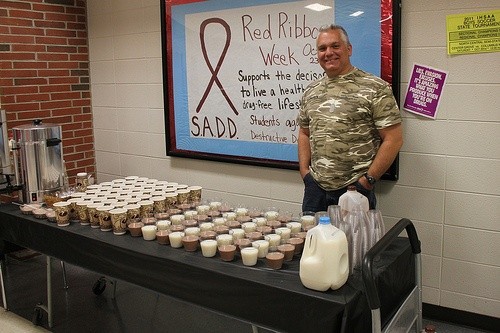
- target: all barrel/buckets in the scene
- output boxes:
[0,110,12,185]
[13,119,67,204]
[338,186,370,211]
[298,218,349,291]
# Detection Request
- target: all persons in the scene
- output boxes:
[296,24,403,216]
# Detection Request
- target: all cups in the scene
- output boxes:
[315,205,385,277]
[44,173,316,270]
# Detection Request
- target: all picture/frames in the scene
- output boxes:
[160,0,402,181]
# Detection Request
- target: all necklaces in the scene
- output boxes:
[324,66,354,113]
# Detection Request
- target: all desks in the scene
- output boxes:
[0,200,421,333]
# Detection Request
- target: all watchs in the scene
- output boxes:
[364,173,377,185]
